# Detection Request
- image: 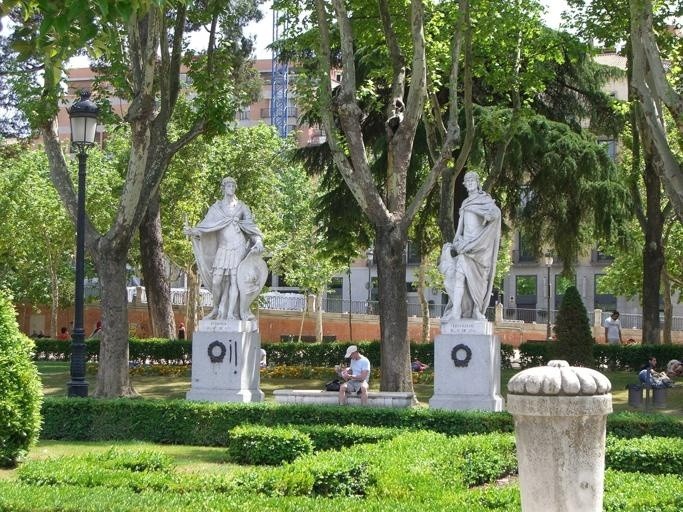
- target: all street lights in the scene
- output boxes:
[365,252,374,297]
[544,252,554,340]
[67,92,99,398]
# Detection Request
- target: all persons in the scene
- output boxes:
[605,311,624,344]
[178,322,185,340]
[339,345,371,406]
[639,357,683,388]
[444,171,501,320]
[411,360,426,370]
[59,327,71,339]
[183,177,264,320]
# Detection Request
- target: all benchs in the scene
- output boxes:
[272,390,412,408]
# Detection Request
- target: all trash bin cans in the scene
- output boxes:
[652,383,666,405]
[625,384,644,406]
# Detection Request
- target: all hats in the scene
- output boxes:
[344,345,357,358]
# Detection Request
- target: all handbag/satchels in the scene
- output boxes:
[325,379,340,391]
